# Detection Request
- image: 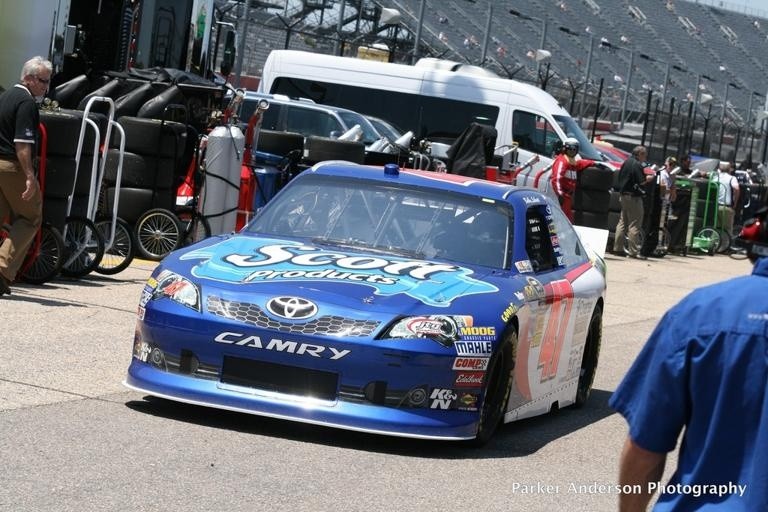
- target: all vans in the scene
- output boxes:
[227,89,424,162]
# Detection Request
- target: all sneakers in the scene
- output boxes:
[0,273,11,296]
[613,250,628,257]
[632,254,647,261]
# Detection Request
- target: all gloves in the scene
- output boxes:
[559,195,564,205]
[597,162,609,170]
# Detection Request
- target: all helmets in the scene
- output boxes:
[564,138,580,151]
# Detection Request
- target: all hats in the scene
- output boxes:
[666,156,678,165]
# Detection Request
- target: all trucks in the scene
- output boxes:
[257,44,606,210]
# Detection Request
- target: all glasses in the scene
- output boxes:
[39,78,50,84]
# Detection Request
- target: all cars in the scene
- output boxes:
[120,150,611,453]
[589,137,757,184]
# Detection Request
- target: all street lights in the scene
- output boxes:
[496,9,766,161]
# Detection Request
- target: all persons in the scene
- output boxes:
[550,137,608,225]
[648,155,678,257]
[726,157,767,186]
[711,160,739,254]
[612,144,653,258]
[0,55,55,297]
[607,256,767,512]
[678,154,693,176]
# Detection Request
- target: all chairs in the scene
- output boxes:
[435,212,506,270]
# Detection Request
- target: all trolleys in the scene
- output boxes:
[621,171,765,259]
[0,82,214,296]
